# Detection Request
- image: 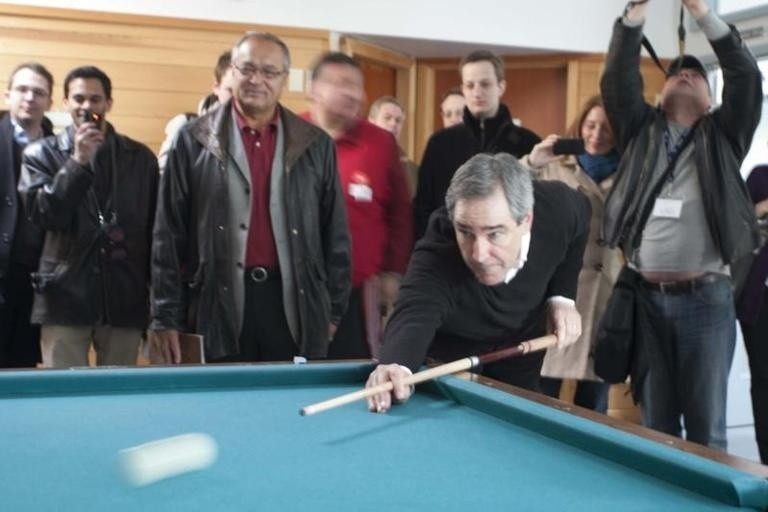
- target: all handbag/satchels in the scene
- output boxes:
[594,266,638,384]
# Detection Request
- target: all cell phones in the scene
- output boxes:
[86,109,102,129]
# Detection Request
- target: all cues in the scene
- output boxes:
[298,334,556,416]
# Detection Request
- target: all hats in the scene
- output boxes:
[666,55,708,75]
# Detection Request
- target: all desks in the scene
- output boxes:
[0,356,767,510]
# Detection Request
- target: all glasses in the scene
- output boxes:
[237,65,285,80]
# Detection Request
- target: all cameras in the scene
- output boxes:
[553,138,584,155]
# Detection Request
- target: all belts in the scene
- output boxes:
[243,267,277,283]
[643,273,726,295]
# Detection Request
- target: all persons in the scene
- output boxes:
[515,95,626,415]
[363,151,591,413]
[368,96,422,197]
[439,90,468,127]
[414,51,542,243]
[735,163,768,466]
[153,33,352,363]
[294,53,414,357]
[1,61,54,368]
[158,51,234,177]
[598,0,762,455]
[19,66,159,367]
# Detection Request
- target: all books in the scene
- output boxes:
[154,330,206,365]
